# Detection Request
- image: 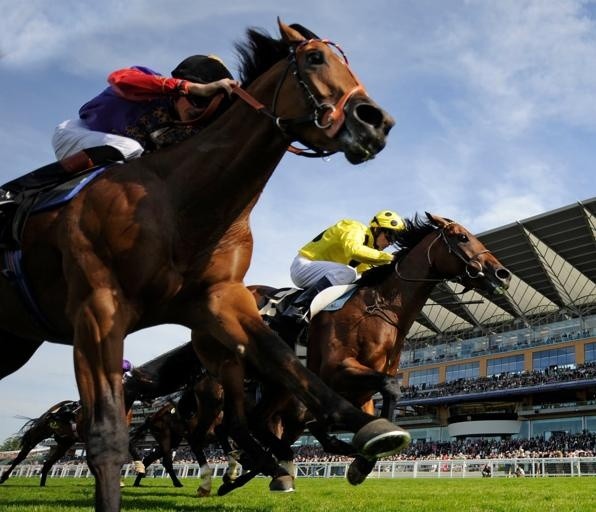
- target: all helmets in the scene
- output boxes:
[368,209,405,234]
[170,52,234,87]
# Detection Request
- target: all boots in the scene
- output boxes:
[0,144,127,214]
[288,273,334,327]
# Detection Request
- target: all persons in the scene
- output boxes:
[282,211,405,325]
[0,54,242,253]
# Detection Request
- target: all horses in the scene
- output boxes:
[0,211,513,497]
[0,15,397,512]
